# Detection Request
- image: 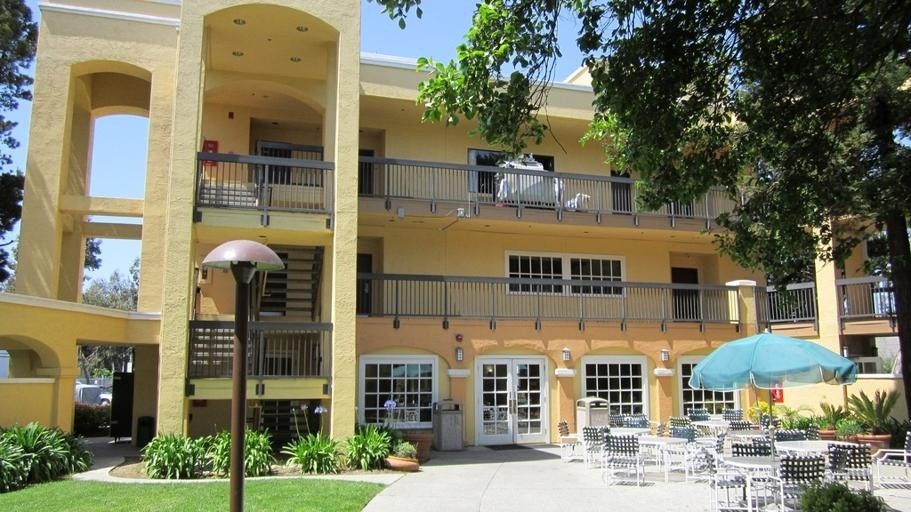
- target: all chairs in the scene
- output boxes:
[583,427,603,467]
[709,432,876,512]
[559,419,583,463]
[873,431,911,486]
[595,408,818,487]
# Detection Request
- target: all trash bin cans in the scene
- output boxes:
[137,416,154,448]
[576,396,610,440]
[432,398,464,451]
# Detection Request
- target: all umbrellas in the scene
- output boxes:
[689,328,858,506]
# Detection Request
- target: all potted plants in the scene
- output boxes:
[817,388,902,454]
[384,440,420,472]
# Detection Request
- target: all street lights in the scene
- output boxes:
[202,239,285,512]
